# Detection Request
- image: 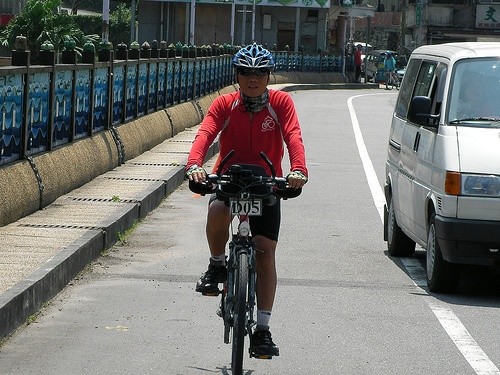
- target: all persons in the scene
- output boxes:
[383,50,400,86]
[183,42,309,360]
[354,44,364,83]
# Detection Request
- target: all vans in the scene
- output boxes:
[384,41,500,297]
[353,42,407,83]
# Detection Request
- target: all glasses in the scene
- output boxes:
[238,68,268,77]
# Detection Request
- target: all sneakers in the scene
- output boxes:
[252,329,279,356]
[195,256,229,293]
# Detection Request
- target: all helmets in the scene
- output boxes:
[231,43,276,70]
[384,50,392,55]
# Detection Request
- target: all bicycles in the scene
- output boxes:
[382,68,400,90]
[188,148,302,375]
